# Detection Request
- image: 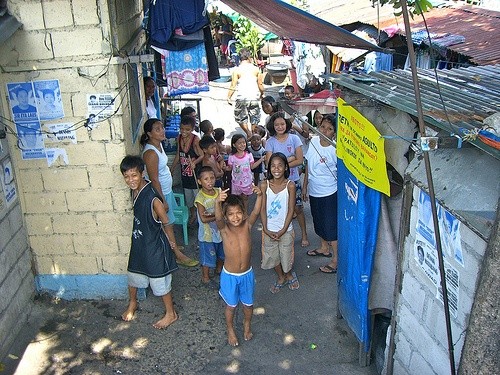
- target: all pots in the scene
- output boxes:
[265,60,288,75]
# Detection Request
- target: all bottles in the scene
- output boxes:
[266,169,266,187]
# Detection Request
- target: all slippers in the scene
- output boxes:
[270,279,286,294]
[319,264,337,274]
[176,258,200,267]
[306,248,332,257]
[286,270,300,290]
[177,245,185,251]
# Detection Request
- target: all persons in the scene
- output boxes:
[193,166,225,290]
[90,114,95,121]
[260,152,299,294]
[139,118,200,267]
[215,186,262,346]
[89,95,98,105]
[169,85,324,224]
[38,89,62,117]
[12,88,36,113]
[121,155,175,329]
[228,49,264,139]
[265,111,310,247]
[300,115,338,272]
[217,49,264,73]
[144,76,157,118]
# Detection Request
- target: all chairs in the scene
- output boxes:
[172,193,189,245]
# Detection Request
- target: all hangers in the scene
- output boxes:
[413,48,428,57]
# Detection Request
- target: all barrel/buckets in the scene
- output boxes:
[166,154,182,186]
[162,115,181,153]
